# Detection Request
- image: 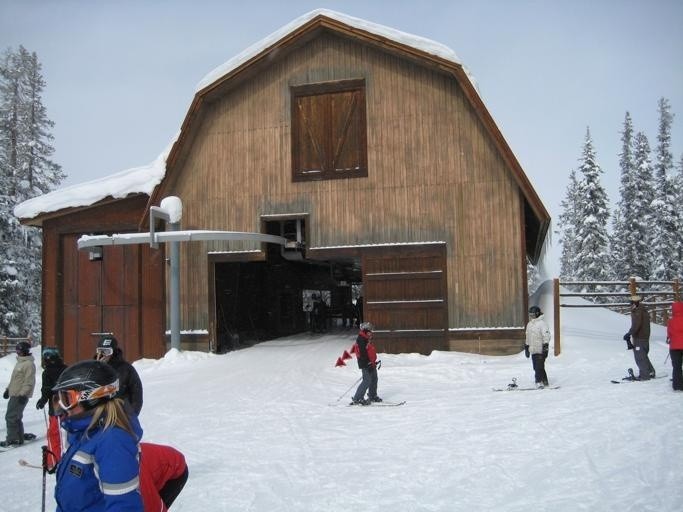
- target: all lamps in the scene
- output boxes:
[86,244,104,262]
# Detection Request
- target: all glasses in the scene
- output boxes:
[96,347,113,356]
[57,378,120,411]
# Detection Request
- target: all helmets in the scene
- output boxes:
[15,342,32,354]
[51,360,124,412]
[95,336,117,354]
[528,306,541,313]
[359,323,374,335]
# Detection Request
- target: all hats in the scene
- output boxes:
[40,347,61,368]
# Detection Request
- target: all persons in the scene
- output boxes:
[666,302,683,390]
[352,322,382,405]
[524,306,551,389]
[305,294,364,334]
[36,347,68,470]
[49,360,144,512]
[0,342,35,447]
[624,295,655,381]
[136,443,188,512]
[94,335,142,417]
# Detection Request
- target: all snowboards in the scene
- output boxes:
[492,384,558,390]
[0,432,47,452]
[610,371,668,383]
